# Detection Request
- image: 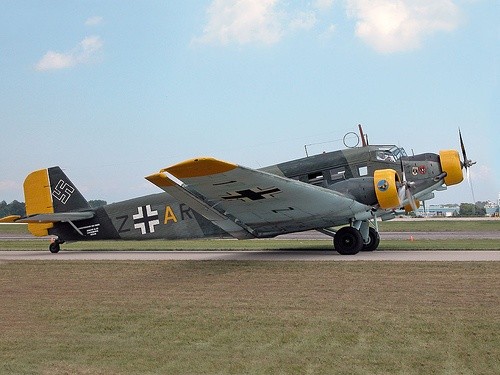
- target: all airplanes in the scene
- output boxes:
[0,122,479,255]
